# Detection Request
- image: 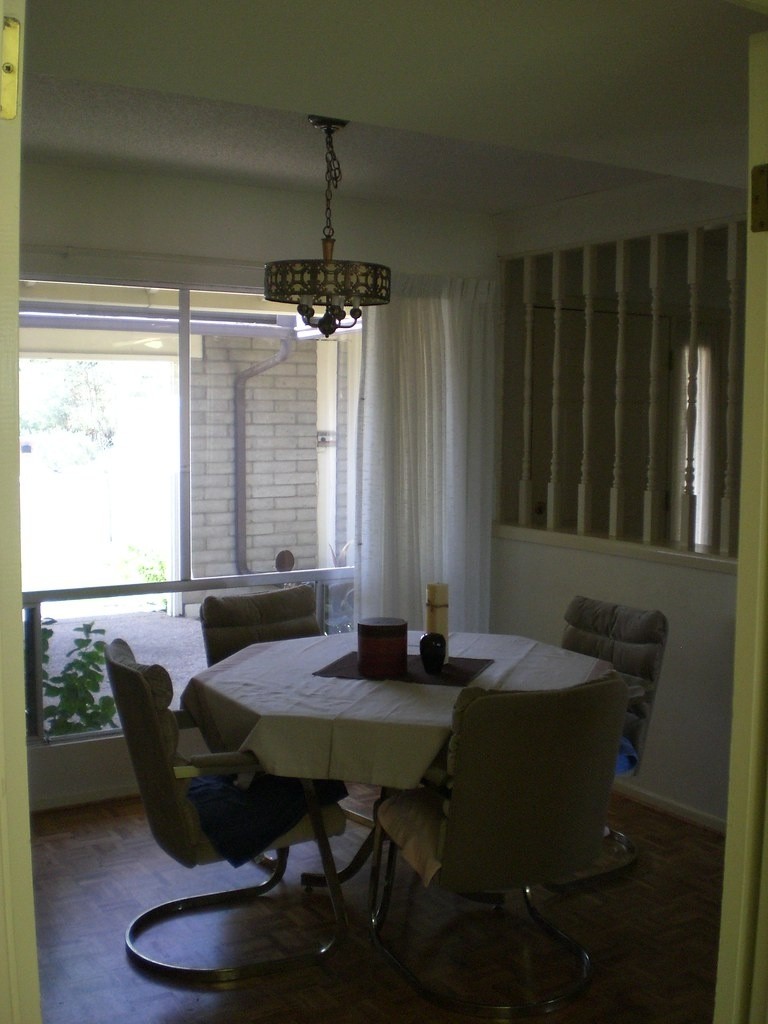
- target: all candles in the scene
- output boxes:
[425,580,450,664]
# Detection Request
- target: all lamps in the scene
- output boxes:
[264,114,392,339]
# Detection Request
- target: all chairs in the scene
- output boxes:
[104,637,349,985]
[366,667,627,1020]
[200,584,375,875]
[535,594,669,894]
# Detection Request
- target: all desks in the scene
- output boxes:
[180,630,613,929]
